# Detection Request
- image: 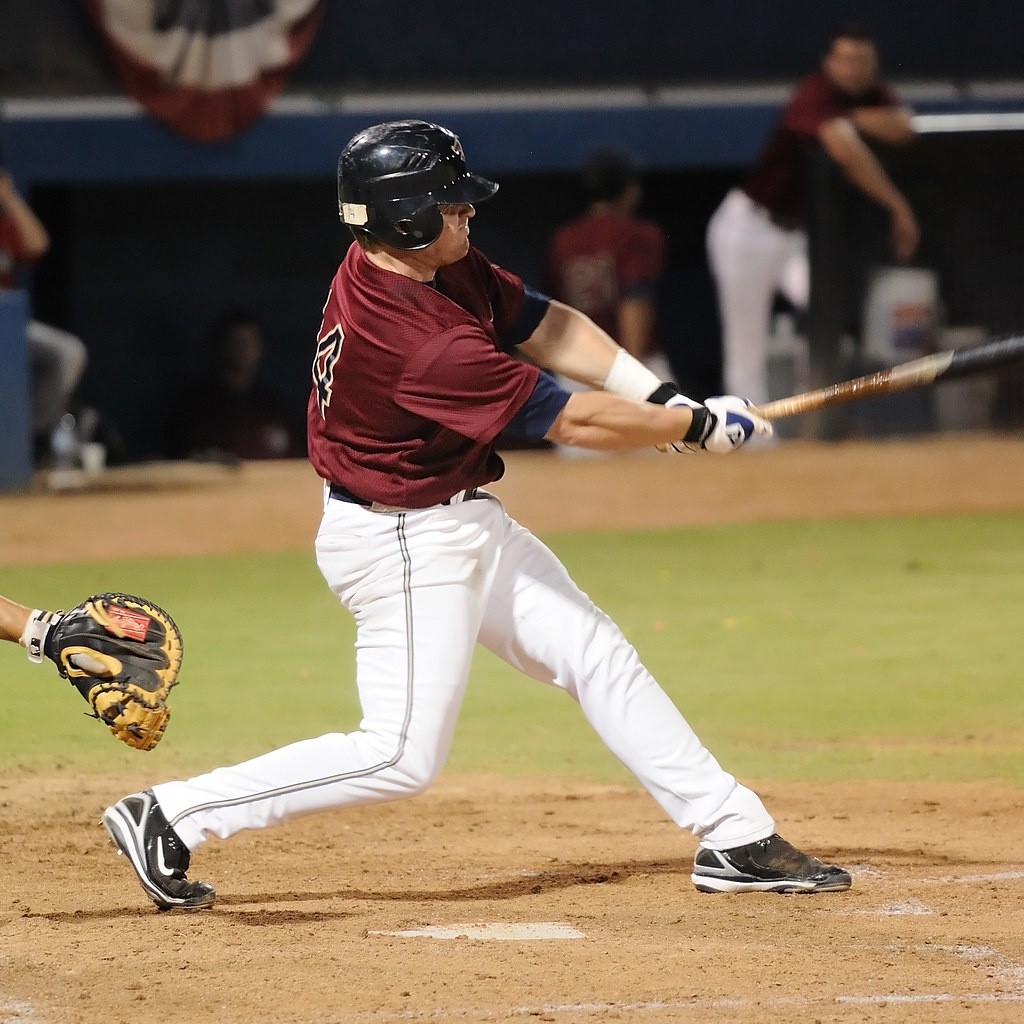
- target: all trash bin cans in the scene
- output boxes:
[0,291,31,492]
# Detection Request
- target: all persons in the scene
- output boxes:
[0,175,47,493]
[554,22,919,458]
[101,120,851,910]
[0,593,183,751]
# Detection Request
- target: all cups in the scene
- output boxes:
[84,442,103,475]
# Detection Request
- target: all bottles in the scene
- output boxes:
[53,413,78,475]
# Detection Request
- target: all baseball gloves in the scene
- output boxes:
[50,591,185,752]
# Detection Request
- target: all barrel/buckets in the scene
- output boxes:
[864,264,936,365]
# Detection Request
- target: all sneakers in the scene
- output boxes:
[97,788,216,908]
[690,833,851,893]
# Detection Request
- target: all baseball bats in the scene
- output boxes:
[652,329,1024,453]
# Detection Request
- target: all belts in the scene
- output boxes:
[329,482,477,506]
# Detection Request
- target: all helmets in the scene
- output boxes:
[336,120,499,251]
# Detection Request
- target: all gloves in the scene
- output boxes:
[646,381,706,455]
[698,395,774,455]
